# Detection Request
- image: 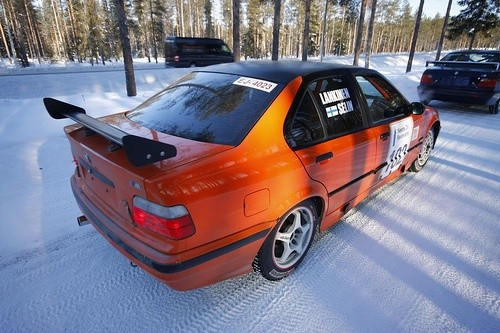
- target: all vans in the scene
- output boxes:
[164,36,235,68]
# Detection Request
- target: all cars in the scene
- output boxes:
[416,48,500,115]
[41,59,444,293]
